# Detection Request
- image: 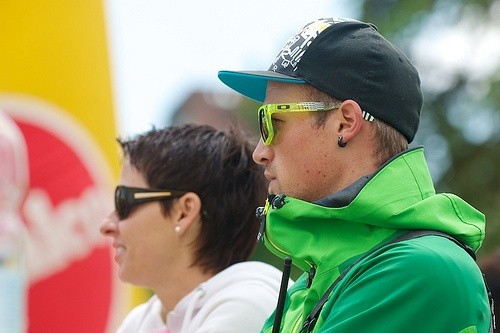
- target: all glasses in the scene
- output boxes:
[114,186,190,220]
[258,99,375,145]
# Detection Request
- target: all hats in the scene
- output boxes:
[218,17,423,143]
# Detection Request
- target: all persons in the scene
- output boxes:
[215,15,494,333]
[98,124,294,333]
